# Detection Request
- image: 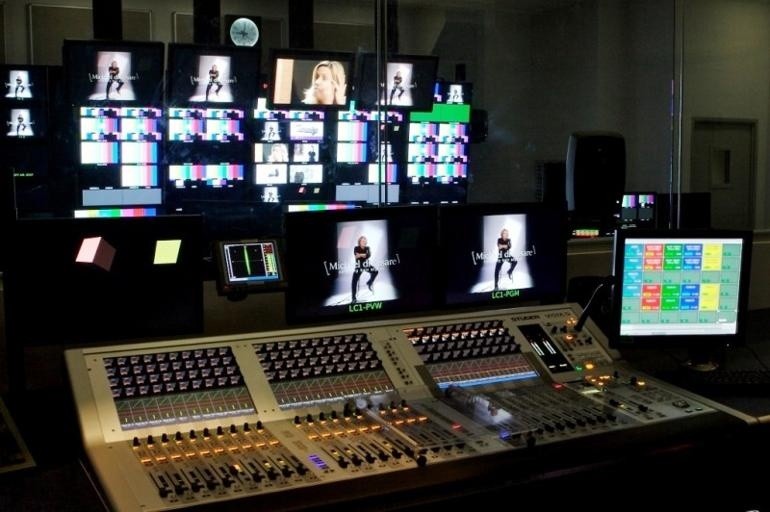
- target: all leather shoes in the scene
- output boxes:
[351,298,356,304]
[508,271,512,279]
[367,280,374,291]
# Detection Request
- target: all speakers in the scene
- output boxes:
[567,132,626,230]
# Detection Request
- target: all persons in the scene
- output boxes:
[389,70,405,105]
[104,61,124,100]
[268,144,289,162]
[453,89,459,100]
[308,146,316,161]
[17,114,26,135]
[301,61,346,105]
[205,64,223,102]
[493,229,517,291]
[15,76,24,97]
[351,236,378,303]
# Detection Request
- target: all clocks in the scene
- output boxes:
[224,14,263,49]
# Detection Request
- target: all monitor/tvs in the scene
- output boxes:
[7,213,205,346]
[61,38,167,111]
[167,42,262,110]
[0,62,489,207]
[623,191,657,230]
[266,48,356,111]
[217,238,284,286]
[609,229,754,350]
[436,200,568,315]
[356,52,441,113]
[286,203,437,324]
